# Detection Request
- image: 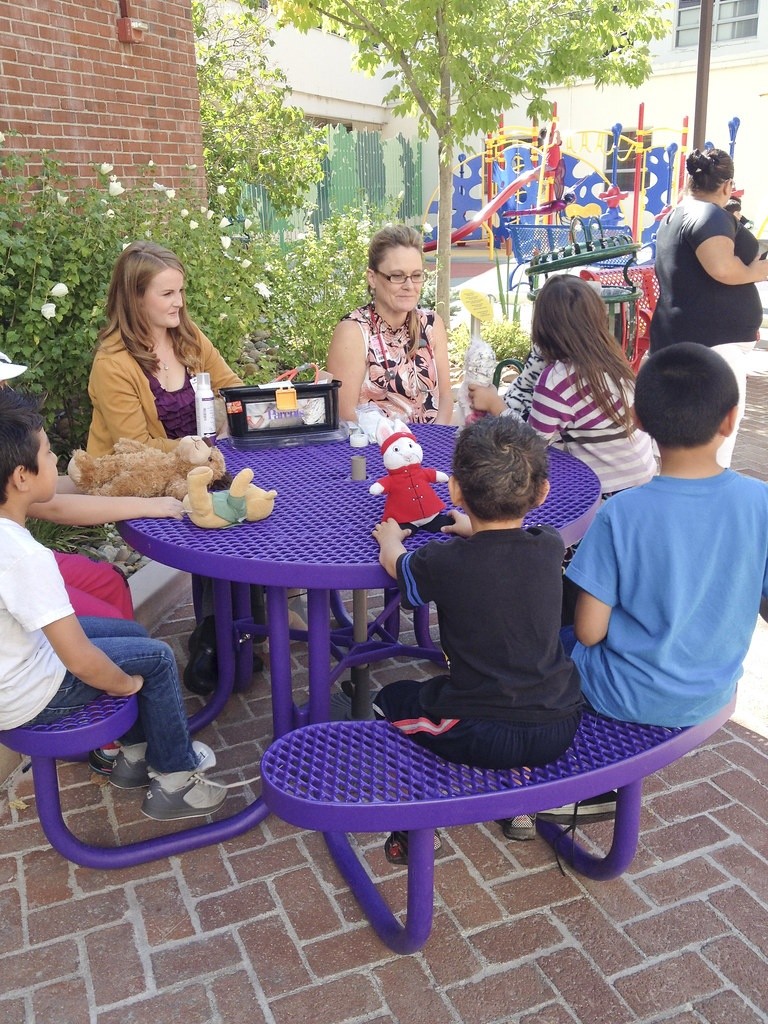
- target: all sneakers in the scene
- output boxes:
[535,790,617,825]
[140,740,229,821]
[111,744,149,789]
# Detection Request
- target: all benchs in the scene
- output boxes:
[260,685,736,956]
[0,695,137,870]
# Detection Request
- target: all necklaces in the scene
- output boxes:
[159,342,171,371]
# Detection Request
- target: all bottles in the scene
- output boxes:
[195,373,217,447]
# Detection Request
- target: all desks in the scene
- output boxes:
[115,423,601,742]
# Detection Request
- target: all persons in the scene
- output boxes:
[0,354,261,820]
[373,416,585,866]
[326,223,454,427]
[472,149,767,826]
[87,241,274,693]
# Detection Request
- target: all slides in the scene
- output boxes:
[423,165,544,253]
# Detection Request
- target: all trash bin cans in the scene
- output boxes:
[525,235,643,358]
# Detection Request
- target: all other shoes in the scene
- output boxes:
[184,616,221,694]
[89,747,115,775]
[384,829,444,865]
[504,813,537,840]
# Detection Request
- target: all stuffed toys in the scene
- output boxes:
[369,419,456,533]
[68,434,277,527]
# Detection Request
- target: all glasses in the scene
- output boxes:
[375,267,429,284]
